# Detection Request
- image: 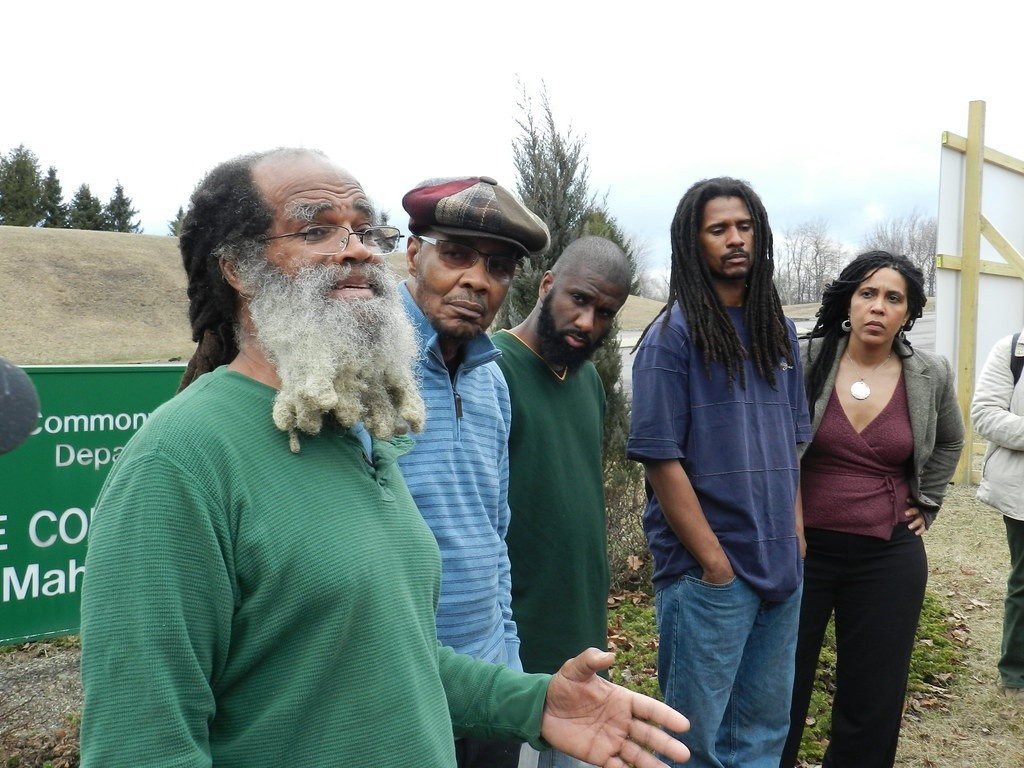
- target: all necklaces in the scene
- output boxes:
[844,350,895,400]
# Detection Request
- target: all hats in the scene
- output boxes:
[0,357,40,455]
[402,175,551,259]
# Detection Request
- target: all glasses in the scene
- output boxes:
[255,225,405,256]
[416,235,524,283]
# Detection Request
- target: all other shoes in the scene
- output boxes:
[1002,686,1024,701]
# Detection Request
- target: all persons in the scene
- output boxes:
[489,236,608,766]
[970,328,1023,700]
[398,176,526,767]
[627,176,812,767]
[79,147,691,767]
[778,251,965,767]
